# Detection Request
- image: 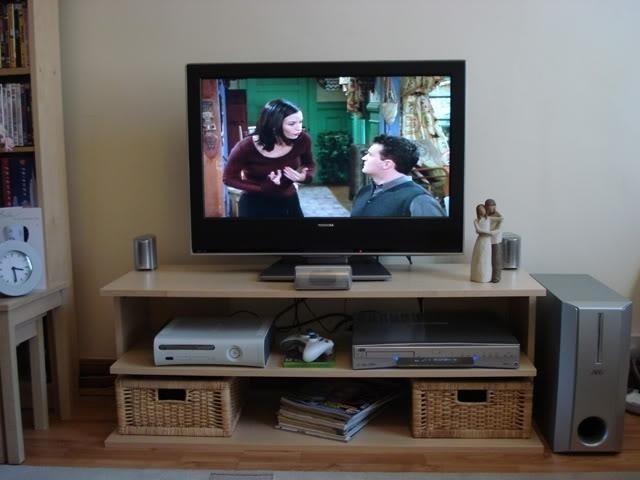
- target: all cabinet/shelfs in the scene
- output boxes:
[98,262,546,455]
[0,0,74,286]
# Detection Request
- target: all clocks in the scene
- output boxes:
[1,240,42,297]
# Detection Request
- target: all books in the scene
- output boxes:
[273,381,408,443]
[0,0,41,208]
[280,347,338,369]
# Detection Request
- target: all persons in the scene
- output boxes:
[483,199,504,284]
[469,204,504,282]
[348,133,447,218]
[223,99,315,217]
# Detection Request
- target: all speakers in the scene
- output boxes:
[134,234,157,270]
[295,265,352,290]
[531,273,632,453]
[500,232,521,270]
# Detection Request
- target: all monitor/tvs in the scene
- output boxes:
[186,59,467,281]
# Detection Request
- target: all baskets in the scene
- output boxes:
[410,379,537,441]
[112,378,244,438]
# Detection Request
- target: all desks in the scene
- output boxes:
[0,288,61,464]
